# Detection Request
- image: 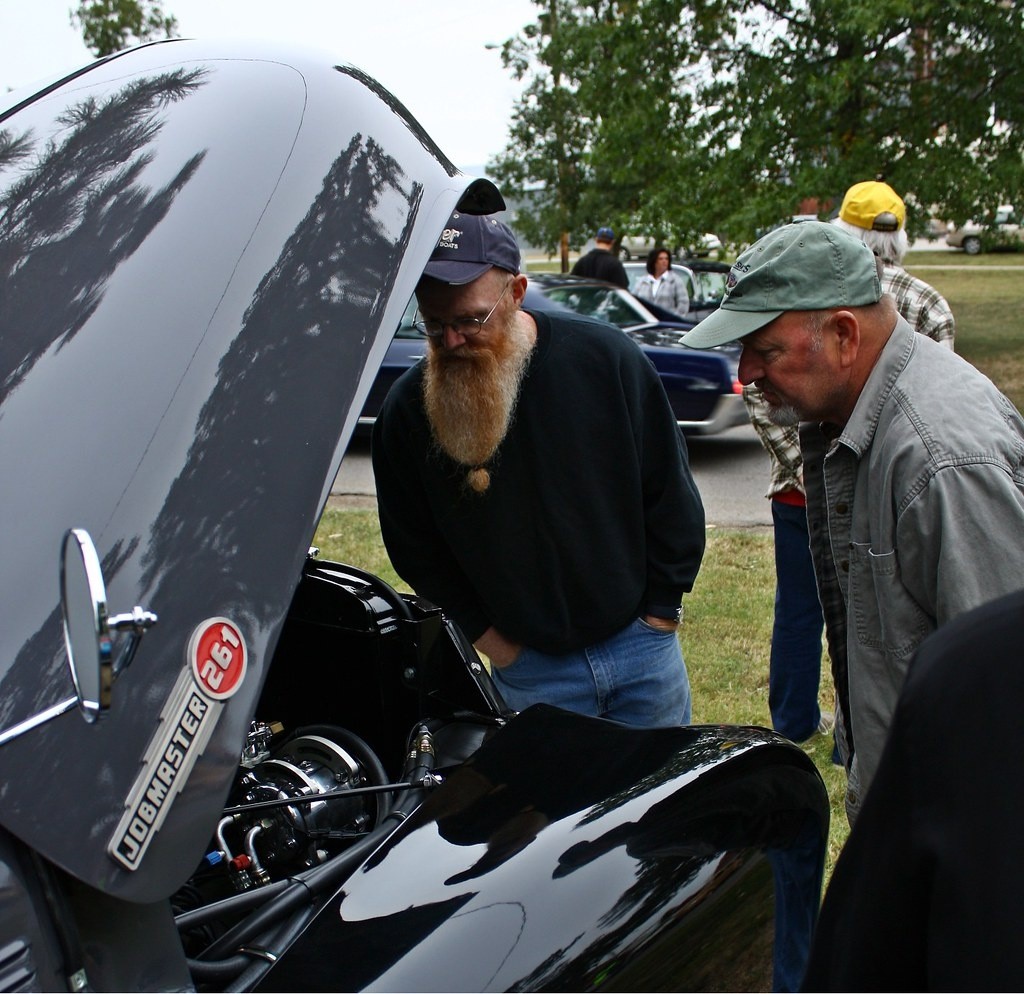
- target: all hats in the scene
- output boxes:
[676,219,884,349]
[421,209,522,285]
[830,180,905,231]
[597,227,614,241]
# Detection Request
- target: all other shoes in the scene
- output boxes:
[795,712,835,748]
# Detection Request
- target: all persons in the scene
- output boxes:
[568,226,629,289]
[741,180,954,769]
[631,246,689,318]
[370,213,705,726]
[678,218,1023,824]
[804,588,1023,993]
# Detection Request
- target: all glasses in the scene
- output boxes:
[411,285,511,335]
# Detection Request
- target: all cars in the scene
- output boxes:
[1,38,830,994]
[945,203,1024,252]
